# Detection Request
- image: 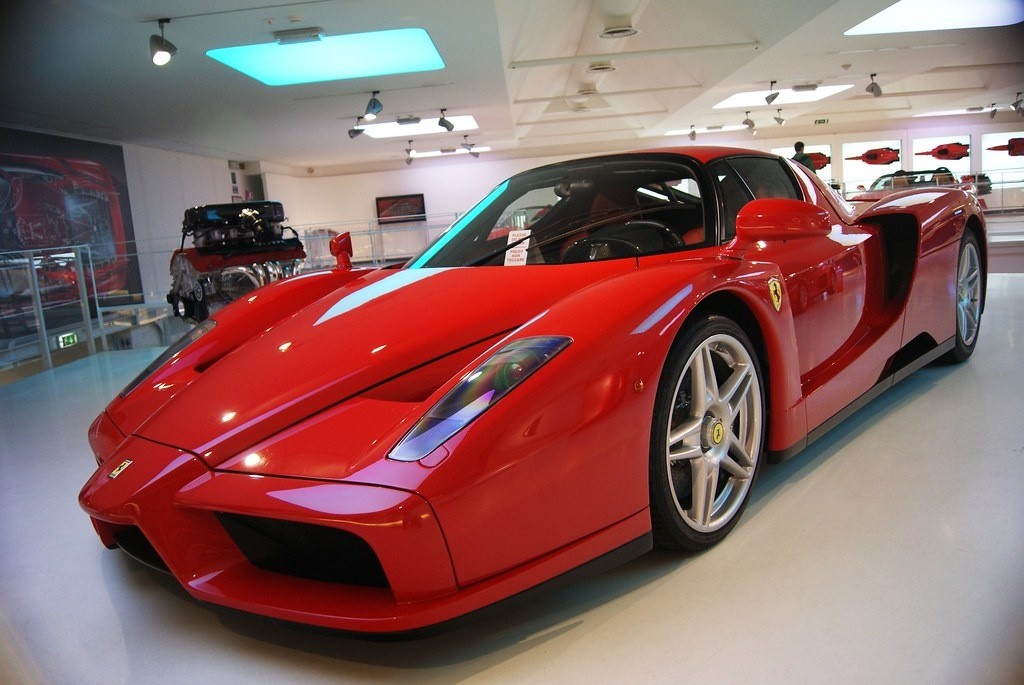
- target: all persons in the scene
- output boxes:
[792,142,815,172]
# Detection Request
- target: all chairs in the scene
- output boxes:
[930,167,954,182]
[889,170,910,188]
[561,190,642,256]
[680,173,767,245]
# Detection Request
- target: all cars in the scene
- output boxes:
[851,167,958,203]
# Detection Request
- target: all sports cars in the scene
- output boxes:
[77,144,990,641]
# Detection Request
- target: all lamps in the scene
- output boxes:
[349,117,364,139]
[774,109,785,125]
[405,157,413,165]
[866,74,882,97]
[461,135,475,151]
[438,109,454,132]
[150,18,177,66]
[364,91,383,119]
[748,128,756,135]
[470,153,479,158]
[405,140,416,157]
[743,111,754,128]
[765,80,779,104]
[688,125,696,140]
[990,103,997,118]
[1010,92,1023,110]
